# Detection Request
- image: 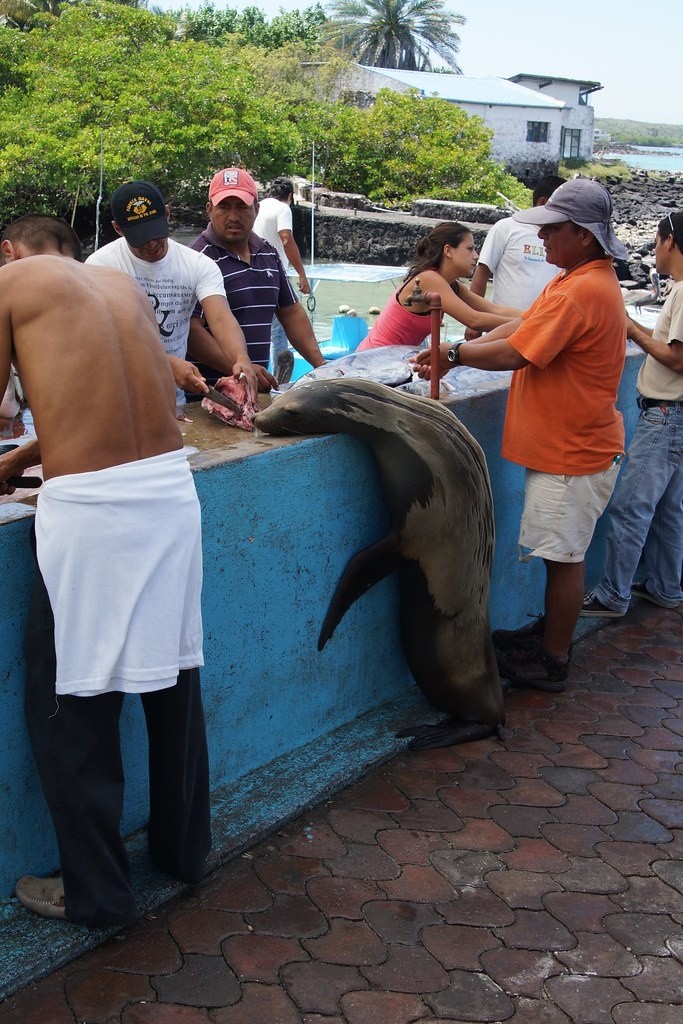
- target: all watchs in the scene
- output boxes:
[447,342,463,367]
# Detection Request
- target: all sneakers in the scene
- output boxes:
[579,592,625,617]
[494,615,575,693]
[630,578,678,609]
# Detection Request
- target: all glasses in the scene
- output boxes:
[665,211,675,248]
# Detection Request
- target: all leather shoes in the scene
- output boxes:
[16,874,66,918]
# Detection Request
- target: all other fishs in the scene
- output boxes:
[394,381,456,397]
[287,345,437,388]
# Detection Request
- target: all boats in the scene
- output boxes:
[266,263,451,383]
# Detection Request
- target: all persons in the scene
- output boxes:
[0,213,213,931]
[82,181,260,411]
[408,178,627,694]
[253,173,311,386]
[468,178,571,342]
[181,168,328,404]
[352,222,527,358]
[575,211,683,618]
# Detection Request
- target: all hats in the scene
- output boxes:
[110,181,171,248]
[511,178,628,261]
[208,168,258,206]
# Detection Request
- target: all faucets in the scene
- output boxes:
[402,276,425,308]
